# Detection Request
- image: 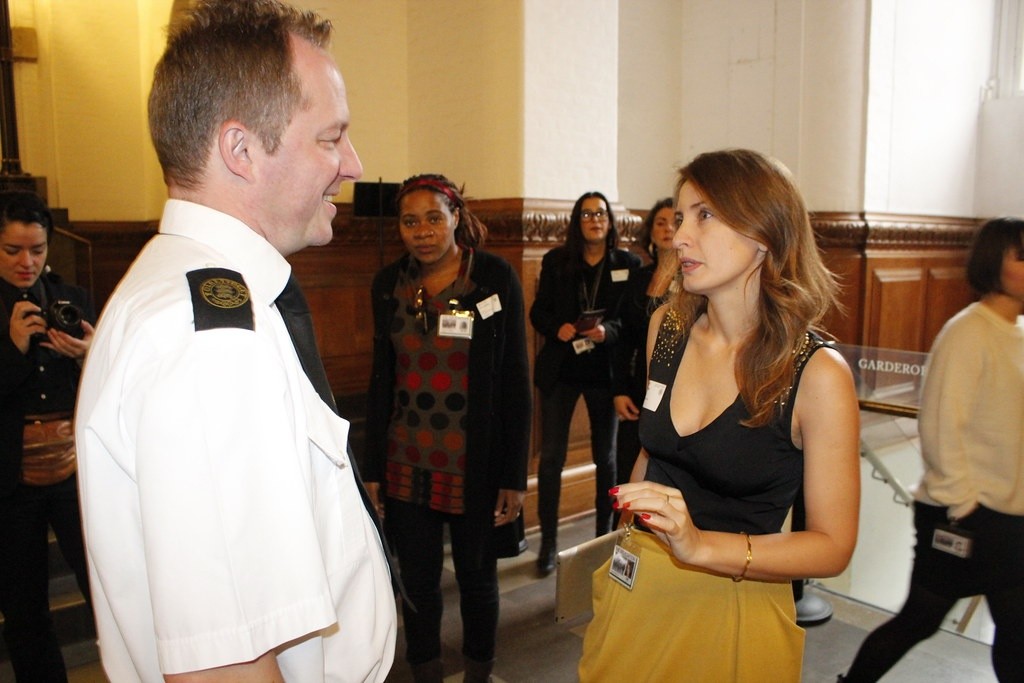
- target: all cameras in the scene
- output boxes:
[32,300,86,342]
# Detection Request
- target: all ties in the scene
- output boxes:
[277,271,418,615]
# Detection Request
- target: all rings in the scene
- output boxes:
[666,494,670,504]
[517,511,521,517]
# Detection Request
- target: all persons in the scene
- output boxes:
[71,0,401,683]
[0,190,95,683]
[529,192,683,579]
[355,171,529,683]
[574,146,863,683]
[833,216,1024,683]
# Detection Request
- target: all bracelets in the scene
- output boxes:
[732,531,753,583]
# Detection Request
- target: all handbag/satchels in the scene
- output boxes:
[20,413,76,486]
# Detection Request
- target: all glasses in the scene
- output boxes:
[579,209,608,222]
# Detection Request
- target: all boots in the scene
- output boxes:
[461,655,498,683]
[408,657,444,683]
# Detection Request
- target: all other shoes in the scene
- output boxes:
[538,534,558,577]
